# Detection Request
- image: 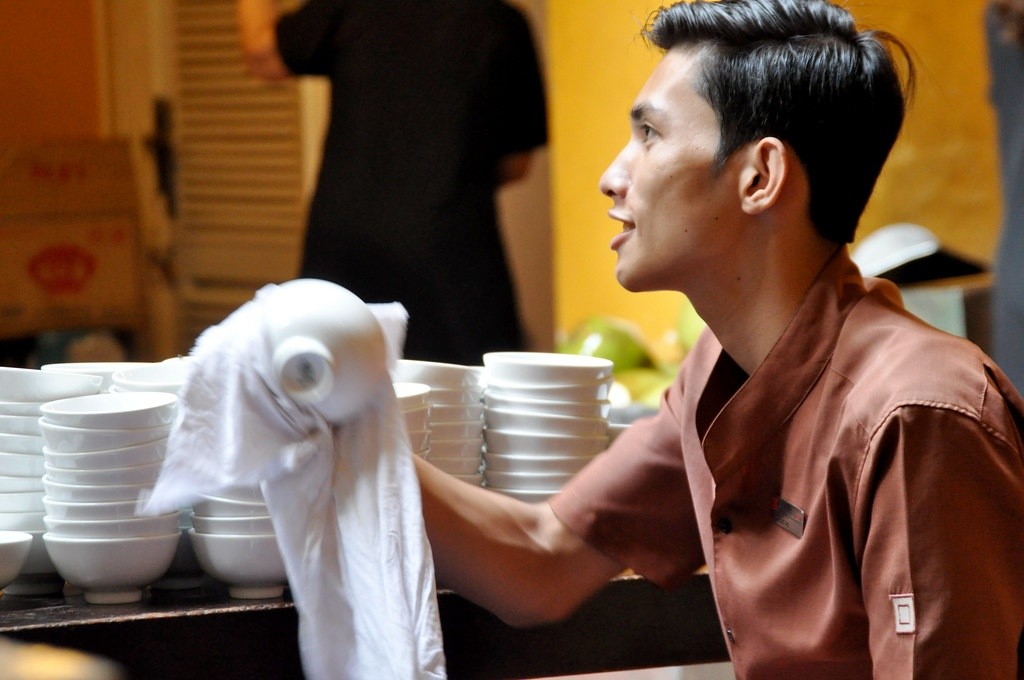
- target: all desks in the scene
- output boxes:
[0,565,710,651]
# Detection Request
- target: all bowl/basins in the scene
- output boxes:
[0,352,635,604]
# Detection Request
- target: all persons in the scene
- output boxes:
[412,0,1024,680]
[274,0,550,365]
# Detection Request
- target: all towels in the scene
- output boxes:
[134,281,448,680]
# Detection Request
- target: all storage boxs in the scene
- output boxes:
[0,0,154,338]
